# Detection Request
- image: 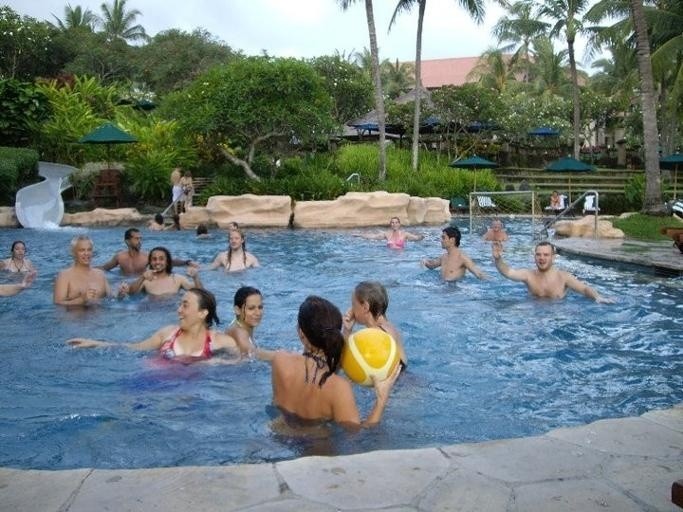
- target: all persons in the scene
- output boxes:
[1,271,38,296]
[148,214,184,232]
[120,246,204,296]
[98,228,200,277]
[551,191,559,210]
[492,240,618,304]
[421,228,483,281]
[53,236,130,307]
[211,228,261,272]
[169,171,194,213]
[272,296,402,428]
[1,240,34,272]
[484,221,506,242]
[342,282,407,383]
[352,217,426,251]
[65,287,241,360]
[195,224,212,241]
[222,285,275,361]
[229,221,238,229]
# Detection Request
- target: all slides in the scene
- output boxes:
[15,161,77,228]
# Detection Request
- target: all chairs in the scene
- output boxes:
[544,195,568,216]
[92,167,124,207]
[581,195,601,215]
[450,197,469,213]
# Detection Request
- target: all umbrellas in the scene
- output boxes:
[447,154,496,191]
[530,128,554,134]
[79,124,137,180]
[542,158,596,204]
[659,152,682,200]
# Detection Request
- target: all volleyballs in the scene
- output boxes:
[340,328,401,388]
